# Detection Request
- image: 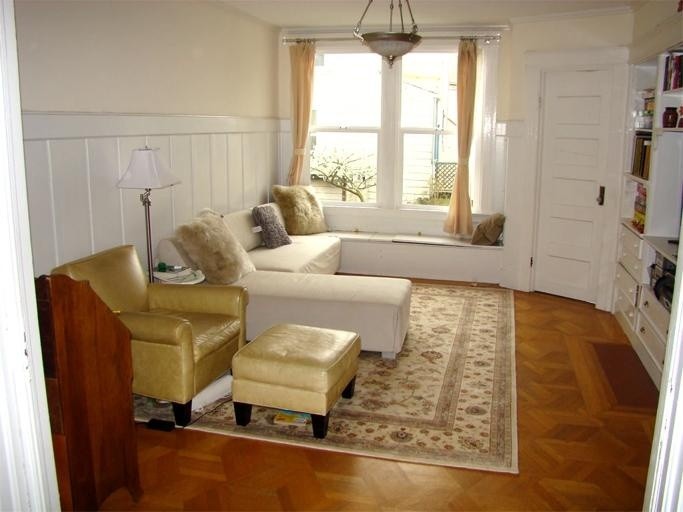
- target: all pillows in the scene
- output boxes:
[470,214,506,246]
[252,206,293,250]
[270,185,329,234]
[174,208,255,284]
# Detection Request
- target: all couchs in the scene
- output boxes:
[164,234,412,364]
[73,245,249,426]
[221,201,341,275]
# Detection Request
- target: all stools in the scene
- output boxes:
[231,322,361,439]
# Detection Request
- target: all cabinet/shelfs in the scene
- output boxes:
[610,44,682,390]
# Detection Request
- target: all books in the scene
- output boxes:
[633,133,652,180]
[663,54,683,91]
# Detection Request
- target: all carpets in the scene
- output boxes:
[130,284,520,474]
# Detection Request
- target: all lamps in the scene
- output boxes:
[353,0,423,69]
[115,147,182,284]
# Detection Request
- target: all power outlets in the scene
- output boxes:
[495,123,506,136]
